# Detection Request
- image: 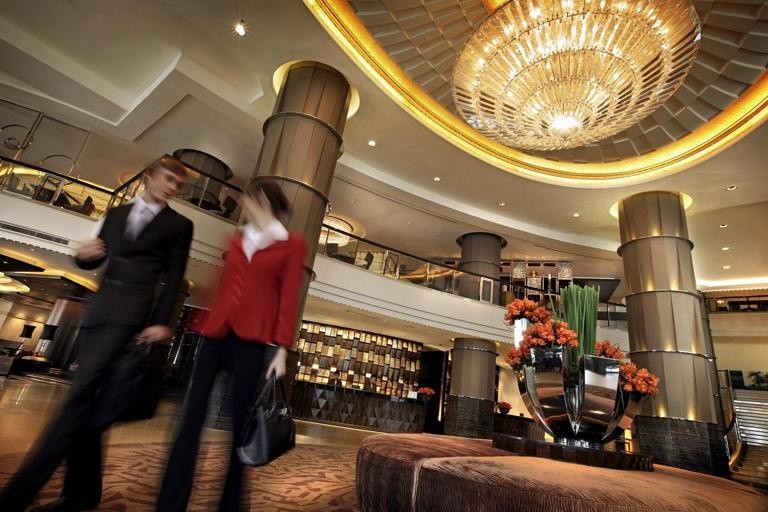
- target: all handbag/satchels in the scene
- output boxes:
[98,352,163,423]
[234,406,295,468]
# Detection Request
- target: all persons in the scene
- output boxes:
[363,374,377,392]
[155,178,308,511]
[327,370,342,386]
[0,158,206,510]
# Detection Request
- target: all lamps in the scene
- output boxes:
[451,0,702,153]
[15,324,58,356]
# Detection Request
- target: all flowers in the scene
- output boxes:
[502,297,579,371]
[594,338,624,359]
[617,362,661,395]
